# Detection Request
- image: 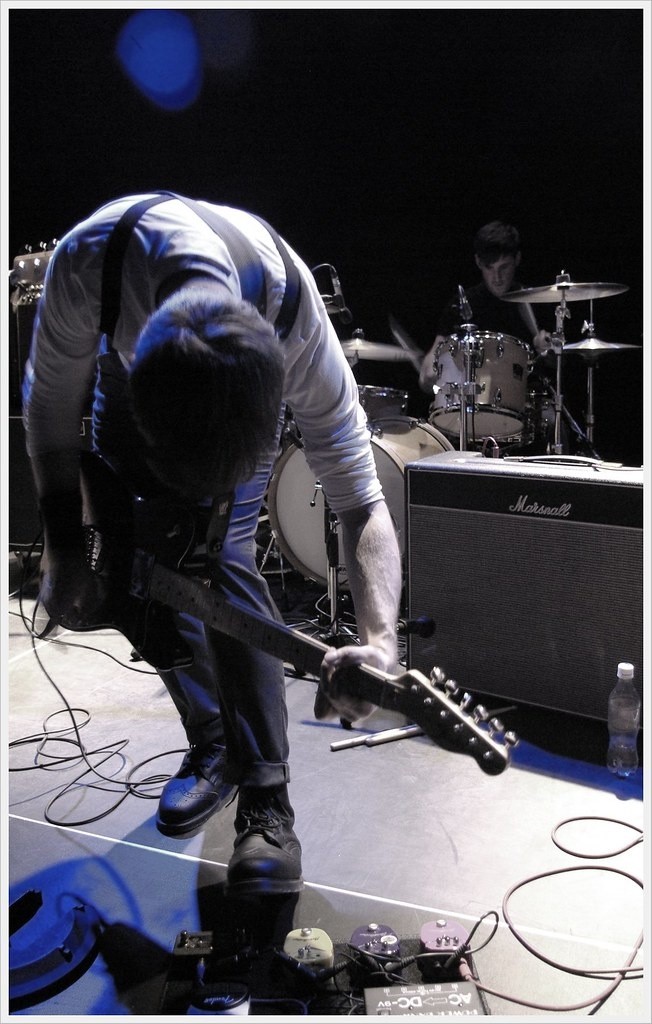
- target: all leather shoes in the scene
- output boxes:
[226,796,302,894]
[156,733,239,834]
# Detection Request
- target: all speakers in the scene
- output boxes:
[403,449,643,734]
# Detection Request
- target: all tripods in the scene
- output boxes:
[289,487,361,645]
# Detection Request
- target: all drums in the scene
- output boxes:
[267,419,461,595]
[427,330,534,443]
[357,383,408,422]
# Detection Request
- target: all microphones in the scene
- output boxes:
[526,350,556,383]
[397,615,437,640]
[330,266,353,325]
[458,286,472,320]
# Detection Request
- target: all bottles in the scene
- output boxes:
[609,661,641,777]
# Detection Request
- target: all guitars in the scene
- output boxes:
[38,448,518,775]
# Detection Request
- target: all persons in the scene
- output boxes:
[421,220,579,430]
[22,188,401,898]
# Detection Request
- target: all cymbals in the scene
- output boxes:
[540,333,642,354]
[499,271,631,303]
[340,329,422,362]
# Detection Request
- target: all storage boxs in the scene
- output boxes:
[401,450,643,760]
